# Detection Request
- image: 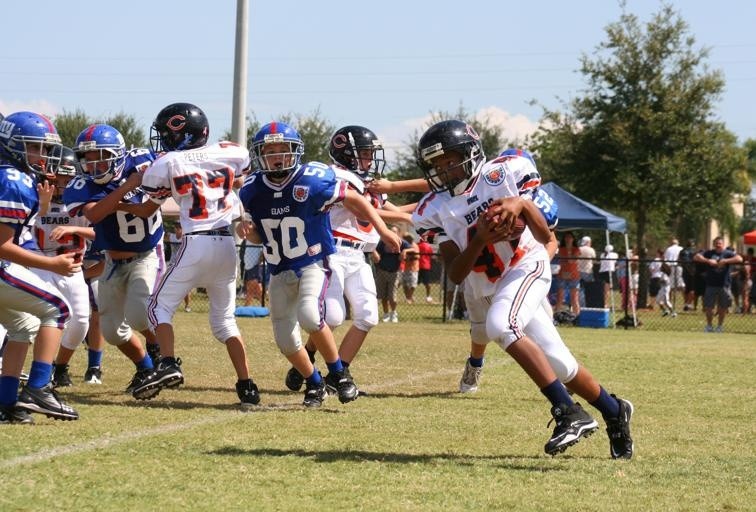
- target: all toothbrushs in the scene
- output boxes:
[482,204,526,240]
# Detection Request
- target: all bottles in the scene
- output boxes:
[149,103,208,152]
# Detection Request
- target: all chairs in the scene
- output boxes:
[84,365,102,385]
[1,361,78,425]
[285,351,360,408]
[602,393,634,460]
[459,358,482,394]
[380,310,399,323]
[127,343,183,399]
[544,401,599,454]
[236,379,260,404]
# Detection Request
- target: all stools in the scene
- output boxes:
[333,237,366,249]
[107,256,133,279]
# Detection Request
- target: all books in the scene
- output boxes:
[577,307,609,328]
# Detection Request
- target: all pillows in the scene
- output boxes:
[250,122,304,176]
[46,146,83,178]
[328,126,386,181]
[1,111,63,184]
[73,124,126,185]
[415,121,485,196]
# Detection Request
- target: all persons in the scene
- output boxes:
[164,222,192,312]
[364,146,561,395]
[240,236,271,308]
[286,125,408,391]
[0,112,165,423]
[113,103,262,410]
[550,229,756,333]
[234,122,402,408]
[410,118,636,462]
[375,226,408,325]
